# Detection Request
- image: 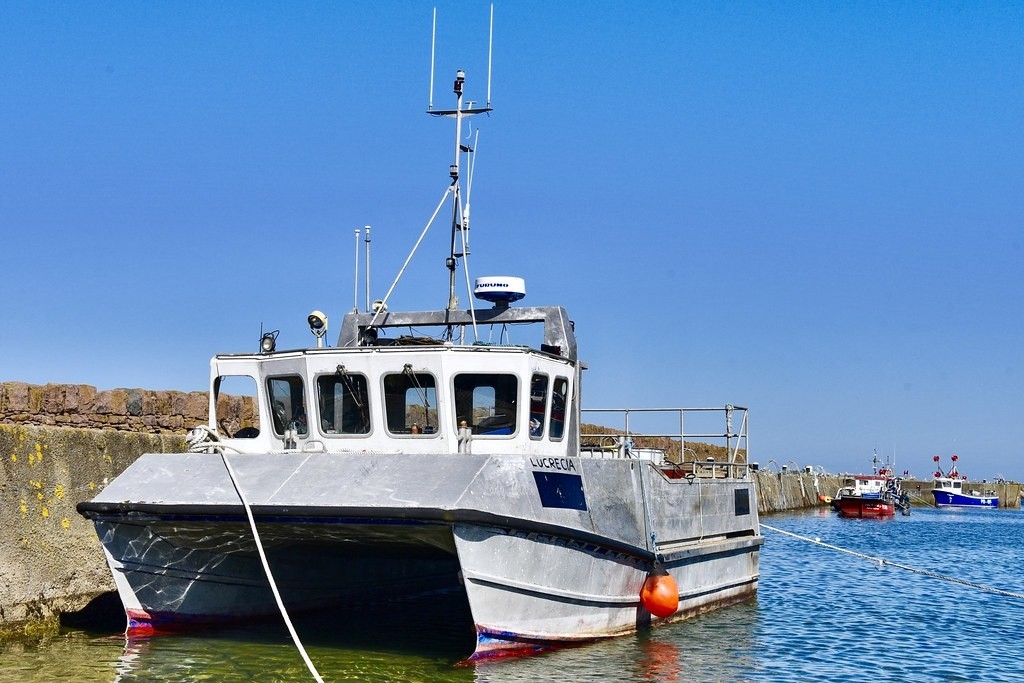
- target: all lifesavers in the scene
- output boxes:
[963,476,966,479]
[885,468,892,477]
[824,496,832,503]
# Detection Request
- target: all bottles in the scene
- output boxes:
[411,423,418,434]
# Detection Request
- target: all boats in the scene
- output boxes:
[68,3,767,669]
[1019,489,1024,509]
[829,447,900,521]
[931,455,999,508]
